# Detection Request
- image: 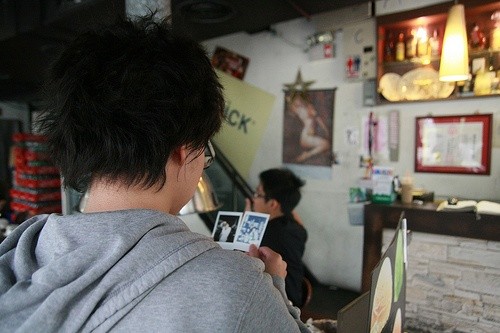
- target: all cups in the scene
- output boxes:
[400,177,412,204]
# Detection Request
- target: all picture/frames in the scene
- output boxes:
[414,111,494,175]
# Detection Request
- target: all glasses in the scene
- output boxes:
[203,138,216,169]
[252,192,267,199]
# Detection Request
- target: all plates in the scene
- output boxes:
[378,67,455,100]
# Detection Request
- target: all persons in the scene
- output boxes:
[1,4,311,333]
[468,24,486,50]
[389,27,441,62]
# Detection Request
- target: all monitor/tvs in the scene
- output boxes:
[336,288,370,332]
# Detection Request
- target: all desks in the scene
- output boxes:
[353,200,500,296]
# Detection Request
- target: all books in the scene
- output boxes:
[436,198,500,220]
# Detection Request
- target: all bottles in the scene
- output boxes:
[406,26,441,58]
[394,28,405,61]
[469,10,500,51]
[384,27,393,62]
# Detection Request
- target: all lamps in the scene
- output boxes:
[438,0,471,82]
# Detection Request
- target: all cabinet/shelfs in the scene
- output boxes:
[373,2,500,106]
[8,133,62,222]
